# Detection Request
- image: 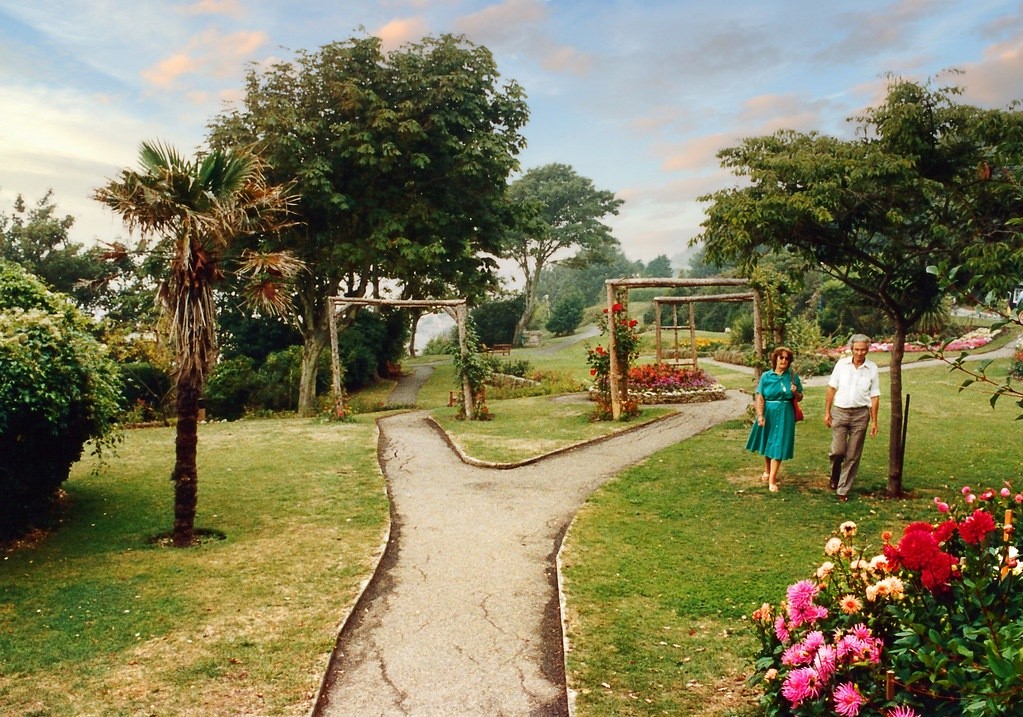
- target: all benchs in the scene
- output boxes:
[482,344,492,356]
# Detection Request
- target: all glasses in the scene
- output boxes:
[778,356,789,361]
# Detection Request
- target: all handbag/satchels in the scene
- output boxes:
[792,371,803,421]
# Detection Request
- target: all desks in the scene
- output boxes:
[492,344,511,356]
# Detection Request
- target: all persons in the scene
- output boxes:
[824,334,881,502]
[744,347,804,493]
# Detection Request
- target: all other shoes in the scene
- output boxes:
[838,493,848,502]
[768,482,779,493]
[762,470,770,483]
[829,476,839,489]
